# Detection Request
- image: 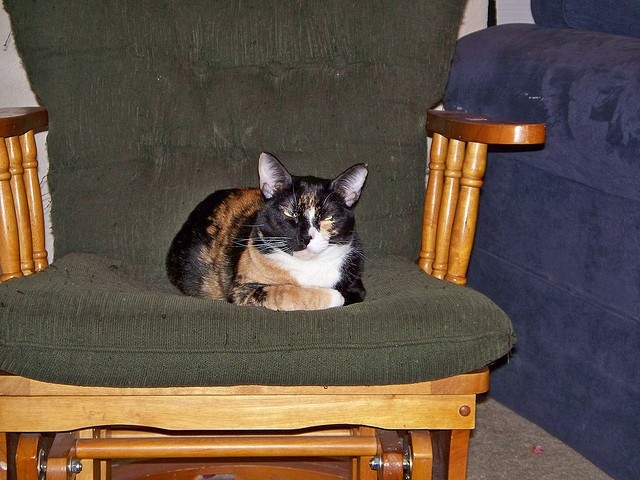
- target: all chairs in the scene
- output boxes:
[0,1,546,480]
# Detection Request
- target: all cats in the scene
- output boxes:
[165,150,369,312]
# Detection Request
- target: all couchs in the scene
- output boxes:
[442,0,640,480]
